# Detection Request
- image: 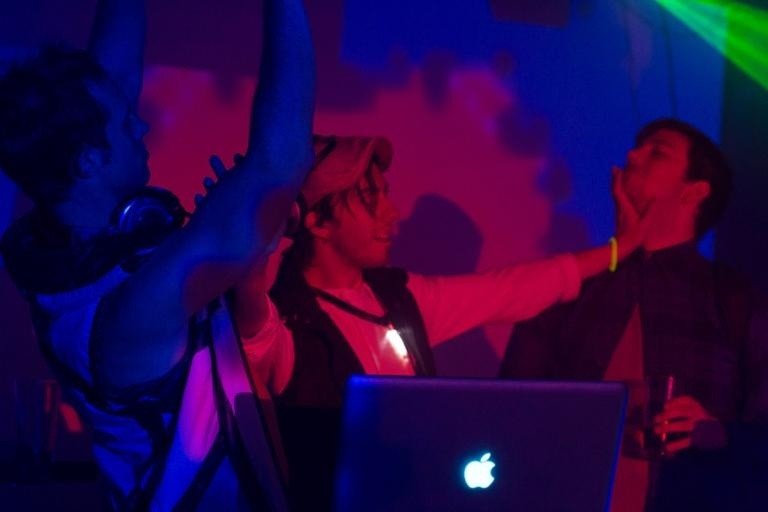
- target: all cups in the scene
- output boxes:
[622,374,685,464]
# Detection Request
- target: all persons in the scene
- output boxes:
[0,2,315,508]
[193,130,663,509]
[497,117,767,512]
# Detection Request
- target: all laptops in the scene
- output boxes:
[328,373,628,512]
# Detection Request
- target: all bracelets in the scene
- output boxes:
[605,238,617,273]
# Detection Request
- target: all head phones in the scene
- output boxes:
[284,194,306,246]
[1,183,185,292]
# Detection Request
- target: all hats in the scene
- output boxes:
[298,133,393,207]
